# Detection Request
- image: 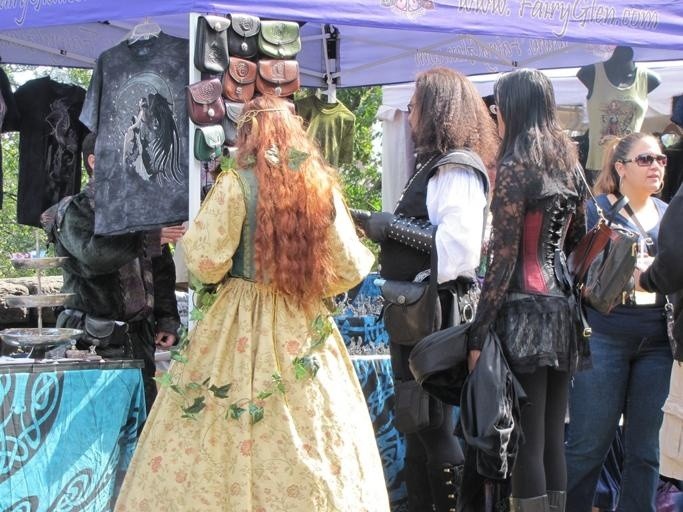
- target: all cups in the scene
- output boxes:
[635,257,656,306]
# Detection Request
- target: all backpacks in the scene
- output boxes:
[564,211,641,314]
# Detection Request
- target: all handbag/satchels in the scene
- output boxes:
[374,275,446,352]
[664,301,683,363]
[185,10,306,164]
[394,375,442,440]
[78,311,131,351]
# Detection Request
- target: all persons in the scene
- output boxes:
[377,65,501,511]
[47,133,186,419]
[576,43,661,184]
[109,91,394,511]
[461,70,590,511]
[562,128,671,512]
[626,178,681,358]
